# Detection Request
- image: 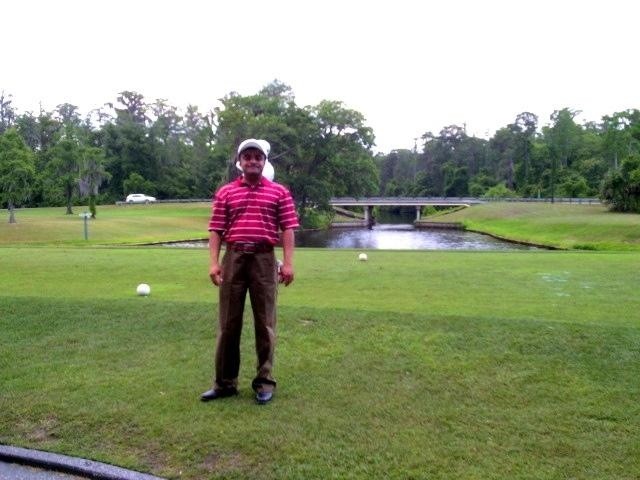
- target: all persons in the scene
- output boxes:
[198,139,301,406]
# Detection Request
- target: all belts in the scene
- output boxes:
[225,242,273,253]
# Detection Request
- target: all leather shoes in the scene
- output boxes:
[199,385,239,403]
[255,392,272,405]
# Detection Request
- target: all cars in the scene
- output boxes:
[126,193,157,204]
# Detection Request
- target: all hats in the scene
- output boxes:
[237,138,267,156]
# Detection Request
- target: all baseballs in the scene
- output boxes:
[358,253,367,262]
[137,284,150,296]
